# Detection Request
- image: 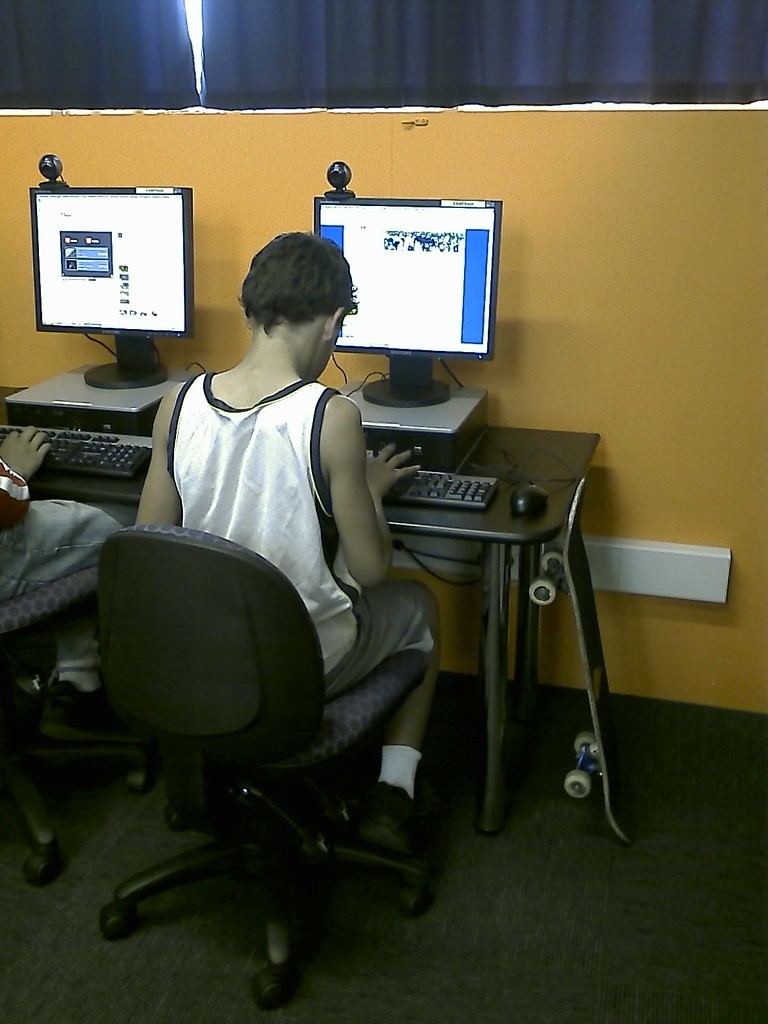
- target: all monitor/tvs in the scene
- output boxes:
[311,196,503,408]
[28,188,196,390]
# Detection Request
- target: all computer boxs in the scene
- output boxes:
[5,360,198,437]
[336,381,489,474]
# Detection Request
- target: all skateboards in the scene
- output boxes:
[533,478,639,851]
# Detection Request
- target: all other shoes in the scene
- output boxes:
[364,782,445,862]
[39,679,135,742]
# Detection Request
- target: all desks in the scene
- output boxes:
[0,386,601,837]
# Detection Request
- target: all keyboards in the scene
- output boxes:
[0,424,154,480]
[391,470,500,510]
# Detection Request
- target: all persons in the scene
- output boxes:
[0,426,151,744]
[134,231,440,856]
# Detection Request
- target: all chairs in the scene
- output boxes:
[0,564,149,888]
[97,523,436,1011]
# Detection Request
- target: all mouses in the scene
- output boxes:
[510,484,548,517]
